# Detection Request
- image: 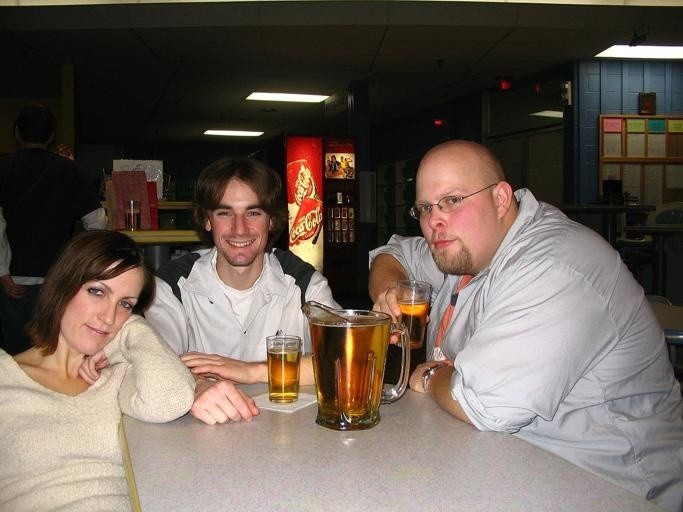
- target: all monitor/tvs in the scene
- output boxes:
[603,179,624,203]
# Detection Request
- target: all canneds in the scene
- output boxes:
[286,159,320,208]
[289,197,324,247]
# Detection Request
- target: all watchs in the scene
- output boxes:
[422,363,447,393]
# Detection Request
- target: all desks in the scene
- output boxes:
[118,378,665,512]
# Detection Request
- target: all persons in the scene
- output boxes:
[133,153,347,425]
[0,100,109,355]
[366,140,683,512]
[0,226,197,512]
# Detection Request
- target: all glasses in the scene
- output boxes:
[409,184,498,221]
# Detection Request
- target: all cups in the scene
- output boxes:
[393,278,433,349]
[266,332,302,406]
[300,302,412,432]
[124,199,141,232]
